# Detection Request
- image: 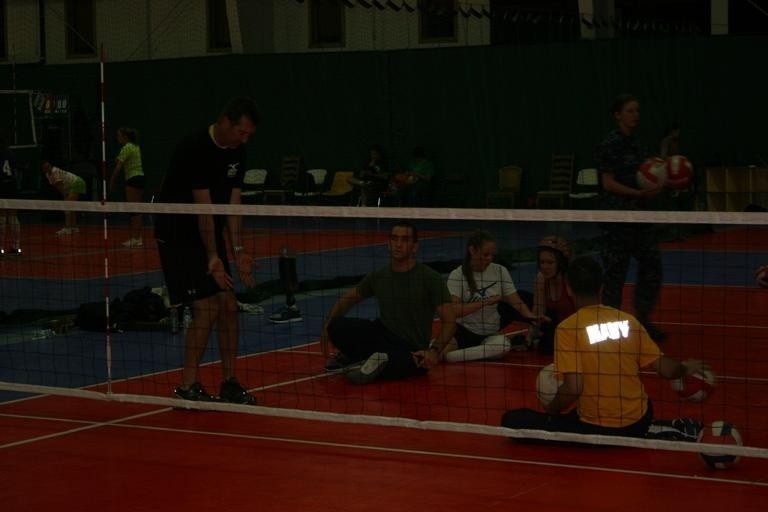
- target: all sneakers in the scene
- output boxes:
[123,236,142,245]
[1,247,21,257]
[172,383,228,410]
[267,307,304,323]
[55,227,79,234]
[219,377,257,406]
[325,351,363,372]
[672,416,704,443]
[510,333,540,353]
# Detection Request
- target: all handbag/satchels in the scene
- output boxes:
[79,287,168,334]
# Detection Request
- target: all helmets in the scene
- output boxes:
[535,235,572,260]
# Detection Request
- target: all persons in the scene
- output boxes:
[151,93,262,412]
[431,227,554,363]
[592,91,691,342]
[347,145,385,206]
[106,125,148,250]
[318,220,458,384]
[521,232,577,355]
[498,255,713,447]
[39,158,89,235]
[0,137,22,259]
[391,148,437,206]
[229,252,304,329]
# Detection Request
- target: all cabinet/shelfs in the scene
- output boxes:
[695,163,768,212]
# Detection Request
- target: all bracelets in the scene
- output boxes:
[230,244,247,256]
[679,363,686,374]
[430,344,442,356]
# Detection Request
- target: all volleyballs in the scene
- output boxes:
[671,359,715,402]
[637,156,692,190]
[697,421,743,470]
[754,264,767,289]
[536,364,566,406]
